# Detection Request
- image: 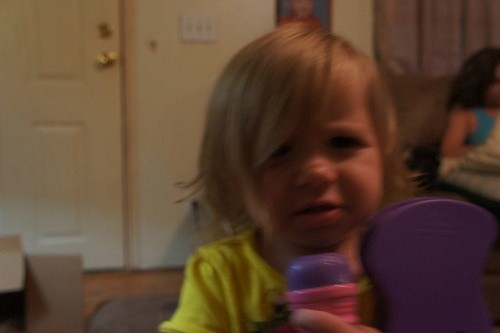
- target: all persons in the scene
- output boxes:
[430,47,499,215]
[156,21,421,333]
